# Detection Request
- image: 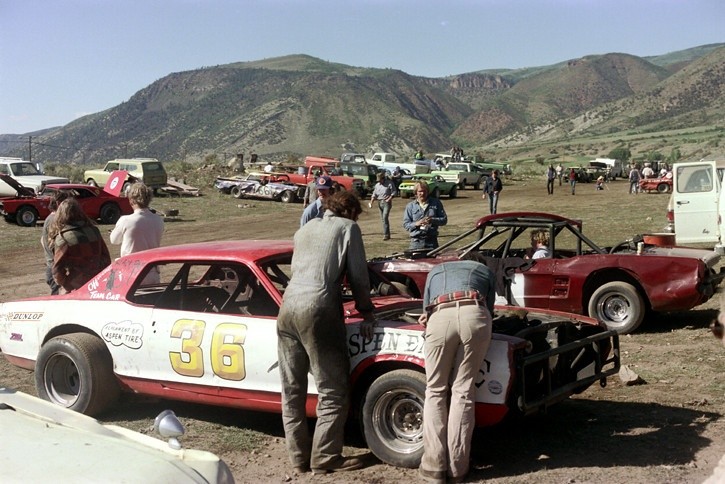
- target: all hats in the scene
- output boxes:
[315,176,332,190]
[376,172,386,181]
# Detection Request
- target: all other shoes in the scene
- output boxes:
[382,235,391,240]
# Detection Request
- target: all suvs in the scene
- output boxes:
[665,161,725,257]
[84,157,168,196]
[0,157,70,197]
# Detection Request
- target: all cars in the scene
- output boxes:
[0,211,720,484]
[0,171,133,227]
[562,166,607,183]
[640,172,673,194]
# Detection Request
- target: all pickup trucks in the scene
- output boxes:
[214,152,508,200]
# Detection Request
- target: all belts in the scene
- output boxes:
[428,300,486,311]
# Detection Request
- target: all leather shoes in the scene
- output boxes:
[418,463,446,483]
[312,458,363,475]
[294,466,307,474]
[447,473,466,484]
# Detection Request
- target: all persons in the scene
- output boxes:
[370,171,398,242]
[332,184,347,197]
[415,249,500,482]
[402,179,449,249]
[329,182,339,192]
[298,175,338,234]
[302,176,321,210]
[271,189,380,474]
[110,181,165,286]
[40,195,69,295]
[543,156,684,196]
[481,170,503,214]
[371,145,466,199]
[46,198,113,295]
[520,231,552,262]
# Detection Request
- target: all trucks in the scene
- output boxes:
[589,158,622,180]
